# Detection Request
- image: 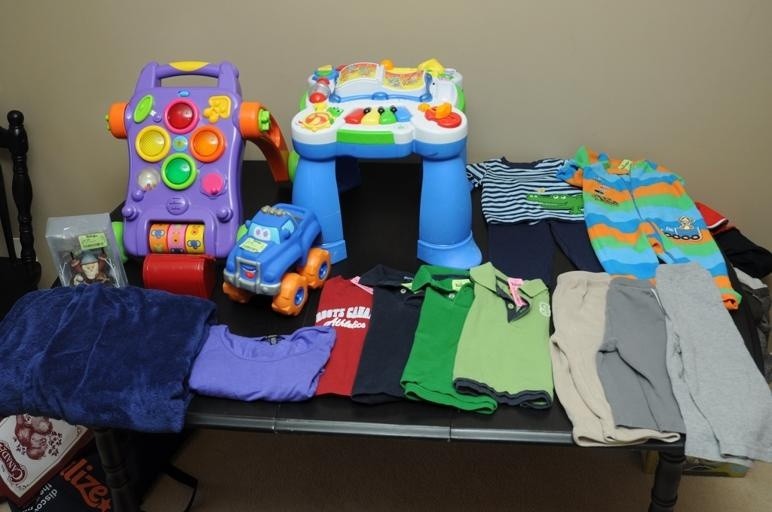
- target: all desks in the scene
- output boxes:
[47,160,688,511]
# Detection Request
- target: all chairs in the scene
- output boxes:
[1,109,44,323]
